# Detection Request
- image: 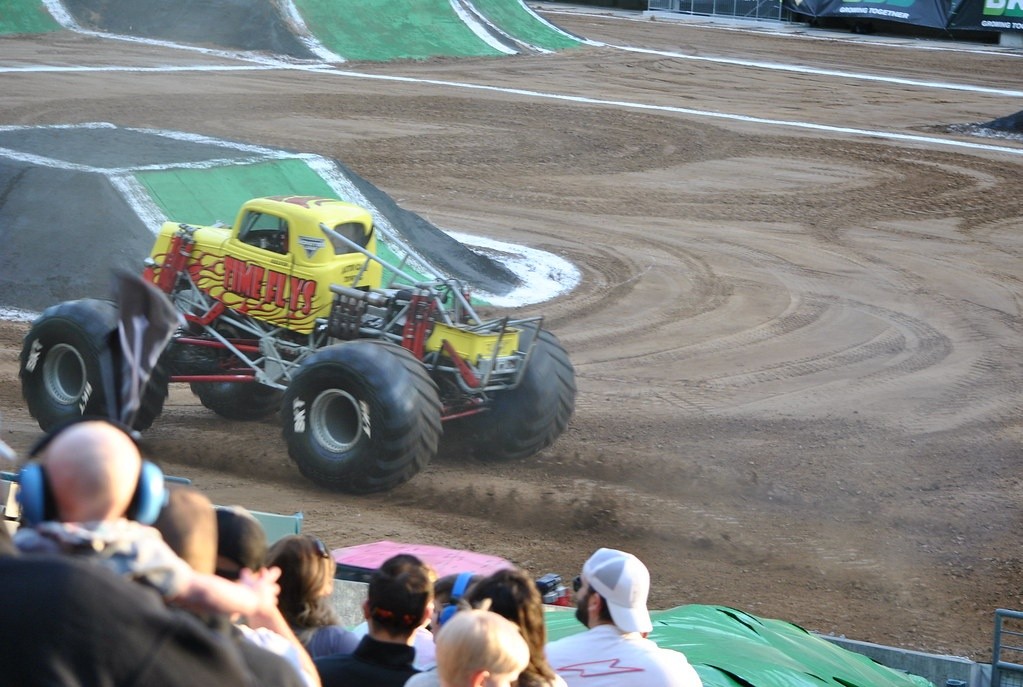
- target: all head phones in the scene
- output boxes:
[15,415,170,526]
[438,572,473,623]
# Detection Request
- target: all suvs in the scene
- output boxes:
[17,193,578,495]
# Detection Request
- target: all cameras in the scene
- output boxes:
[231,562,300,619]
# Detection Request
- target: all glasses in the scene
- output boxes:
[573,576,582,592]
[303,534,329,559]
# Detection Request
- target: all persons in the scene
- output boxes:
[1,416,568,687]
[545,547,704,686]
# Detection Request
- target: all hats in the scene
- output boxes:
[582,547,653,632]
[215,504,265,571]
